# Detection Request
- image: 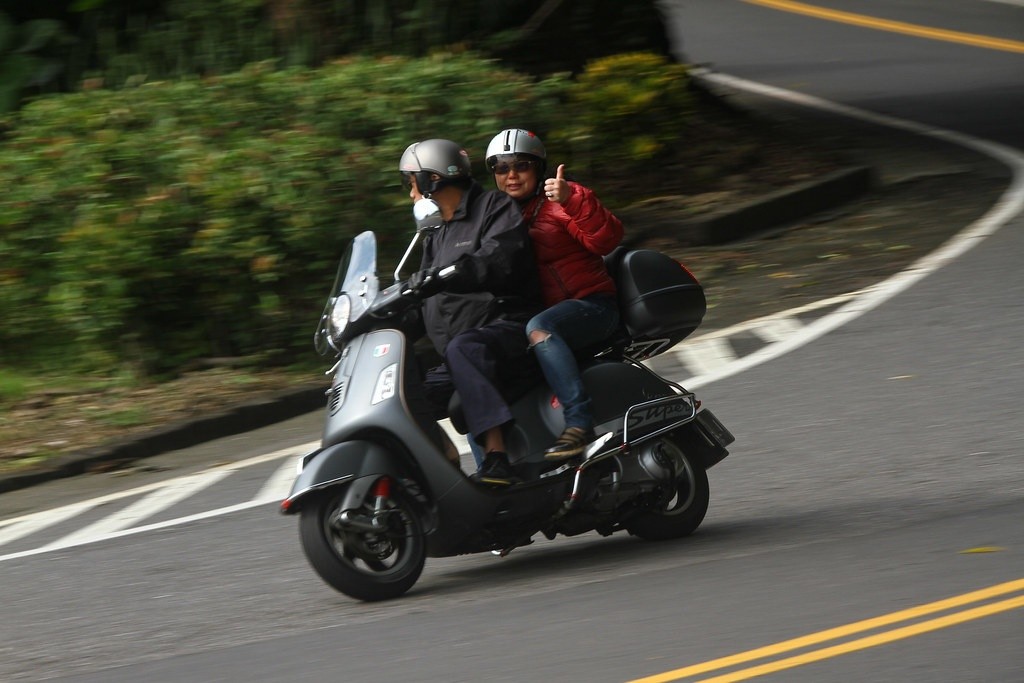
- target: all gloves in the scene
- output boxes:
[409,266,442,298]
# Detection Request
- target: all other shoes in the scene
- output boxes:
[545,423,597,461]
[472,447,512,485]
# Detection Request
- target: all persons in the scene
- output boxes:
[486,129,624,460]
[400,138,543,484]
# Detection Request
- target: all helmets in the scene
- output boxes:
[484,129,547,178]
[397,138,471,194]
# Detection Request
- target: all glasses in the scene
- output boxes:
[489,160,536,175]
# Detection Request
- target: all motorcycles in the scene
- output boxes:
[278,197,735,601]
